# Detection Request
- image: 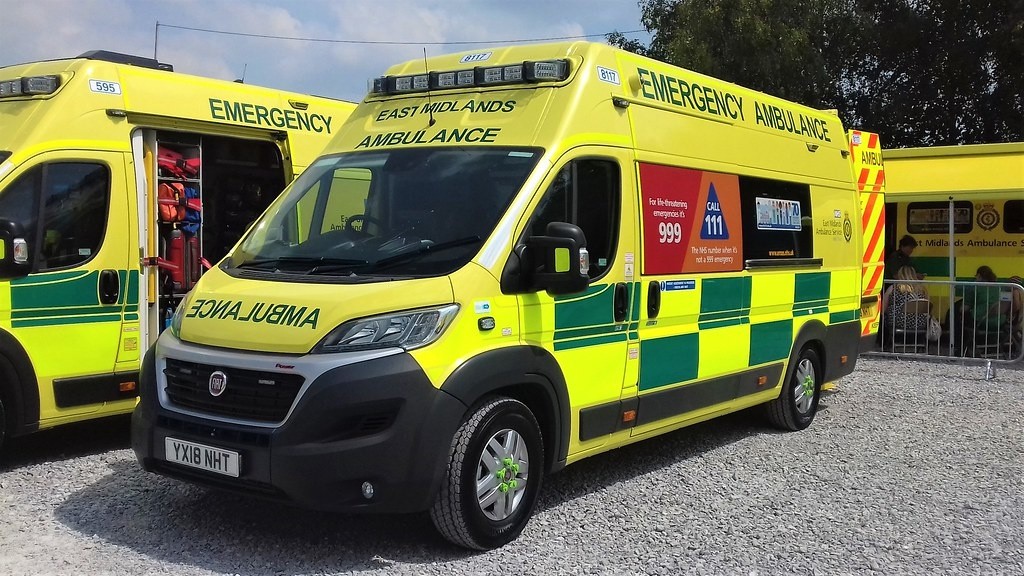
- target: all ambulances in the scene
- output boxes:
[136,39,890,555]
[0,49,374,453]
[882,141,1024,333]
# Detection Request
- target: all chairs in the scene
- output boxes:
[883,299,933,361]
[963,300,1011,359]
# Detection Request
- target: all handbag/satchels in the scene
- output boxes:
[926,318,942,341]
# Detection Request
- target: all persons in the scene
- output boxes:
[879,265,1024,353]
[878,235,920,286]
